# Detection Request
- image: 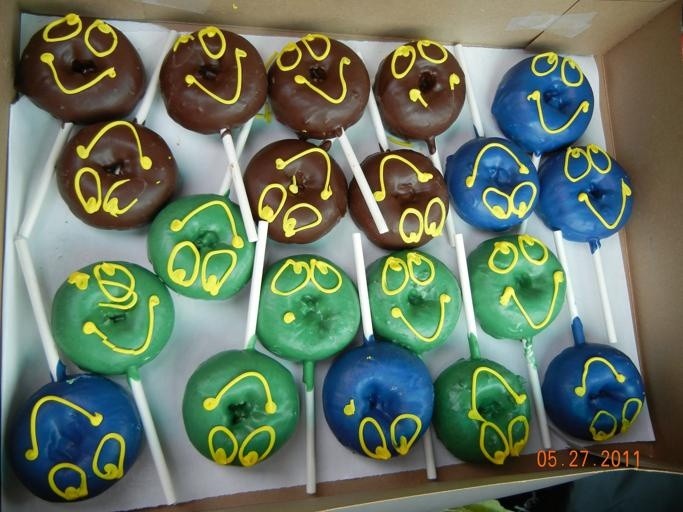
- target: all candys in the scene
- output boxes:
[434,359,532,467]
[491,51,595,156]
[242,139,348,245]
[8,374,144,505]
[52,261,174,375]
[444,136,541,232]
[56,120,177,230]
[542,343,645,442]
[147,195,255,300]
[8,17,147,125]
[321,341,434,459]
[534,143,634,241]
[182,349,299,467]
[467,233,567,341]
[373,38,467,139]
[159,26,267,136]
[349,148,450,251]
[256,254,360,362]
[366,250,463,354]
[269,33,370,139]
